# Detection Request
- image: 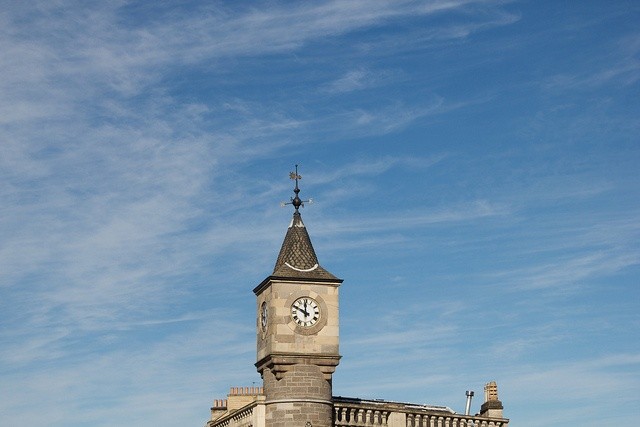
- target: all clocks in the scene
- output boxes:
[284,290,329,337]
[257,299,270,339]
